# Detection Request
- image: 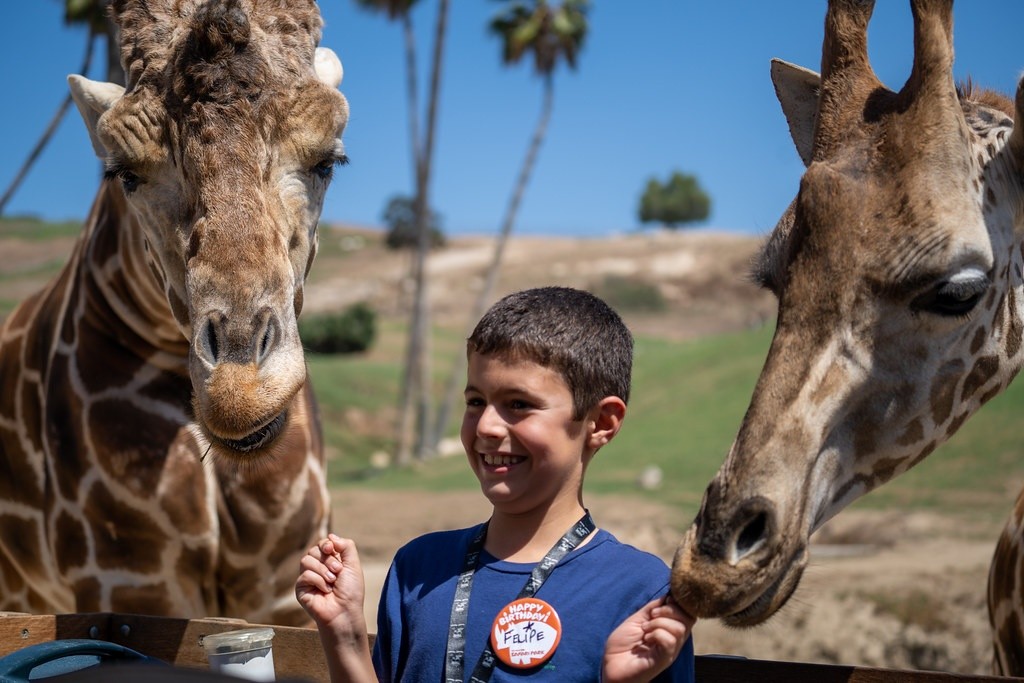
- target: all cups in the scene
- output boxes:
[202,627,276,683]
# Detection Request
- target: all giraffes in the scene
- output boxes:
[1,0,350,631]
[672,0,1024,680]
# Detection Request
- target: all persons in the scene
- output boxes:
[294,285,697,683]
